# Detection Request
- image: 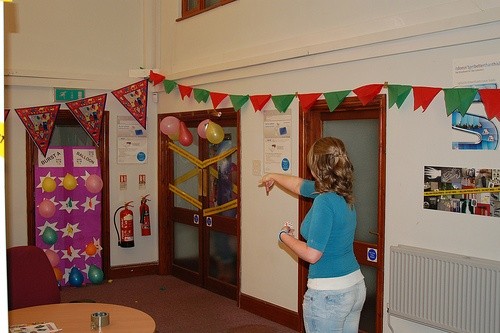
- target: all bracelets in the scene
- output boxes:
[279,232,286,242]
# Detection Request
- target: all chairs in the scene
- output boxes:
[7,245,60,311]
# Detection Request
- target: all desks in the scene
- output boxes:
[8,302,157,333]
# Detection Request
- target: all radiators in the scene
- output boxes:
[386,244,500,333]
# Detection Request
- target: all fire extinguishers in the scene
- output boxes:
[140,194,151,236]
[114,201,134,247]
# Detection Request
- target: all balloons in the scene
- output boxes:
[86,244,96,256]
[206,122,224,144]
[88,265,103,284]
[39,201,55,217]
[46,250,59,267]
[198,119,212,139]
[86,174,103,192]
[42,227,58,244]
[168,131,180,141]
[179,121,193,146]
[161,116,180,135]
[42,177,56,192]
[63,174,77,190]
[69,267,84,286]
[54,268,62,281]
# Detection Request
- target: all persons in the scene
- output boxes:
[214,157,237,263]
[263,137,366,333]
